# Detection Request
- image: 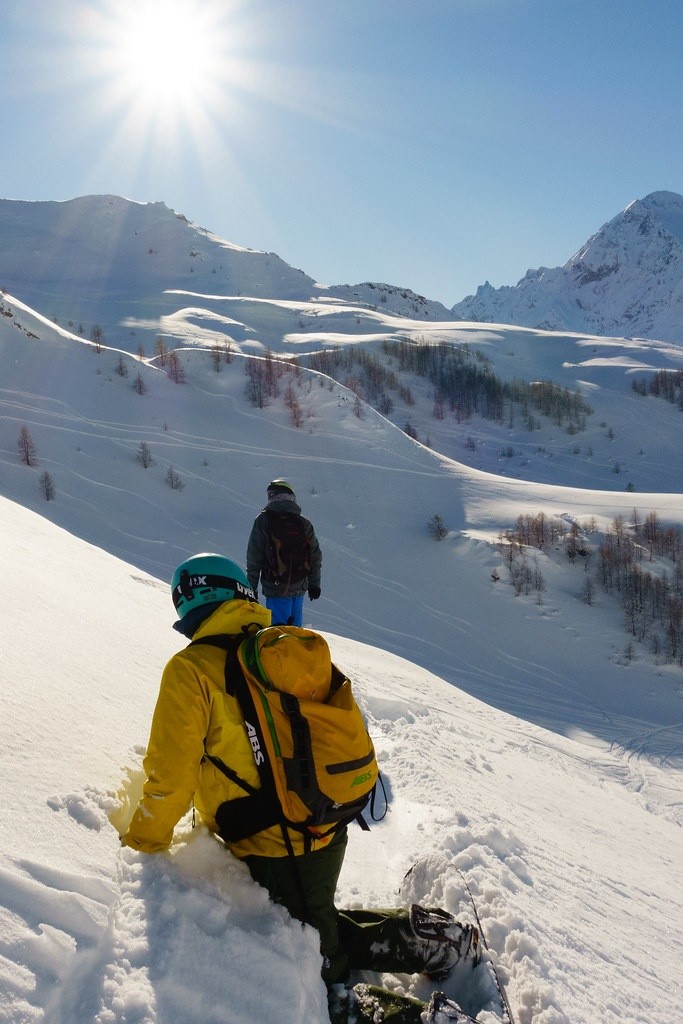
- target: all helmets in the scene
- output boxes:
[267,479,296,502]
[171,553,258,620]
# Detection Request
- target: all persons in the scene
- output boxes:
[245,479,322,628]
[121,553,487,1024]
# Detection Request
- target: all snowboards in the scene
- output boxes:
[399,857,513,1024]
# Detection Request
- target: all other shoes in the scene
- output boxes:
[426,991,484,1024]
[409,902,481,984]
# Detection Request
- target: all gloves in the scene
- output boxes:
[308,585,321,601]
[253,589,259,600]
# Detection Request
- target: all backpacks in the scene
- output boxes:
[185,617,379,826]
[258,511,312,585]
